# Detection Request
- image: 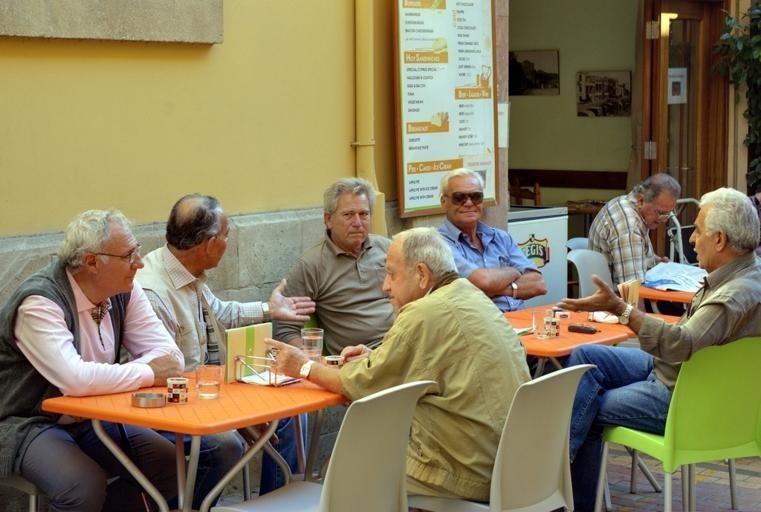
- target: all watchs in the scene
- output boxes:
[618,304,633,326]
[299,360,316,382]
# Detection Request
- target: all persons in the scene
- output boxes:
[433,167,564,382]
[564,188,761,512]
[263,225,534,512]
[268,177,401,365]
[0,206,186,512]
[120,194,316,512]
[586,172,681,313]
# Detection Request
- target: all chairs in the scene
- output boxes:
[595,335,761,510]
[1,473,147,512]
[408,364,598,510]
[214,380,437,510]
[511,179,544,207]
[110,291,252,500]
[564,236,708,309]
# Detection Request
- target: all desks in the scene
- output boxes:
[500,305,679,497]
[43,364,347,510]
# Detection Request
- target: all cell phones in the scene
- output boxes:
[569,323,598,335]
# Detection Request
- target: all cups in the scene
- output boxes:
[300,328,324,363]
[544,317,560,337]
[165,378,189,404]
[533,312,553,339]
[324,354,344,368]
[195,363,222,398]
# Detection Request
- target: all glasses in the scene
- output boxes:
[443,190,484,206]
[95,244,143,265]
[652,204,678,220]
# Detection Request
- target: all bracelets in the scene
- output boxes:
[261,302,270,323]
[511,282,518,299]
